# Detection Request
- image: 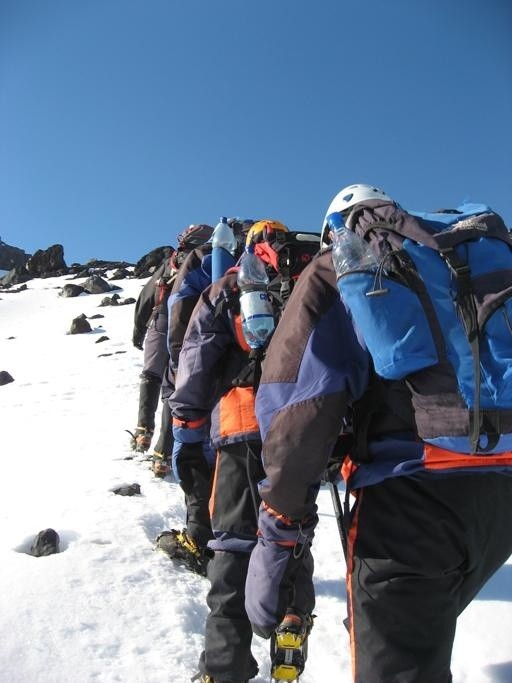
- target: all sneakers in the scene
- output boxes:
[150,451,172,478]
[266,611,310,683]
[157,529,208,576]
[129,425,154,452]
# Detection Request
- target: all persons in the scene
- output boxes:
[152,215,261,579]
[165,216,352,683]
[147,222,215,475]
[133,245,182,454]
[242,182,512,683]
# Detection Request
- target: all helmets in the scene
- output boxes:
[244,217,290,258]
[318,181,395,245]
[176,225,215,247]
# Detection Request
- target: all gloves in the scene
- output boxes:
[245,541,307,639]
[171,440,212,495]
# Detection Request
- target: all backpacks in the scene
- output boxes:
[322,194,512,460]
[220,230,324,382]
[153,252,187,334]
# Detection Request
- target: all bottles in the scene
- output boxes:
[237,247,274,349]
[326,211,388,277]
[211,216,238,255]
